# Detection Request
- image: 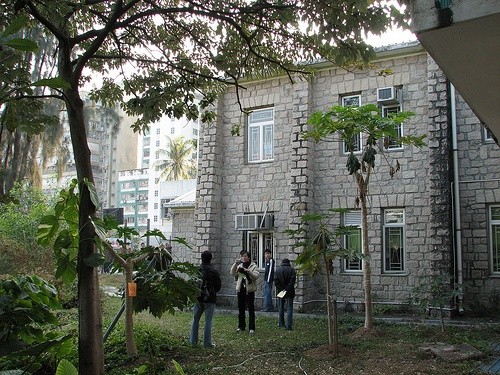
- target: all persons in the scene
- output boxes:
[189,249,221,348]
[261,249,275,312]
[230,251,260,335]
[234,250,247,317]
[274,258,296,330]
[104,236,171,273]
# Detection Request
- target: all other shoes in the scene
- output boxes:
[236,328,241,331]
[249,330,254,335]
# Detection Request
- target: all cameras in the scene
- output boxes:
[236,259,241,265]
